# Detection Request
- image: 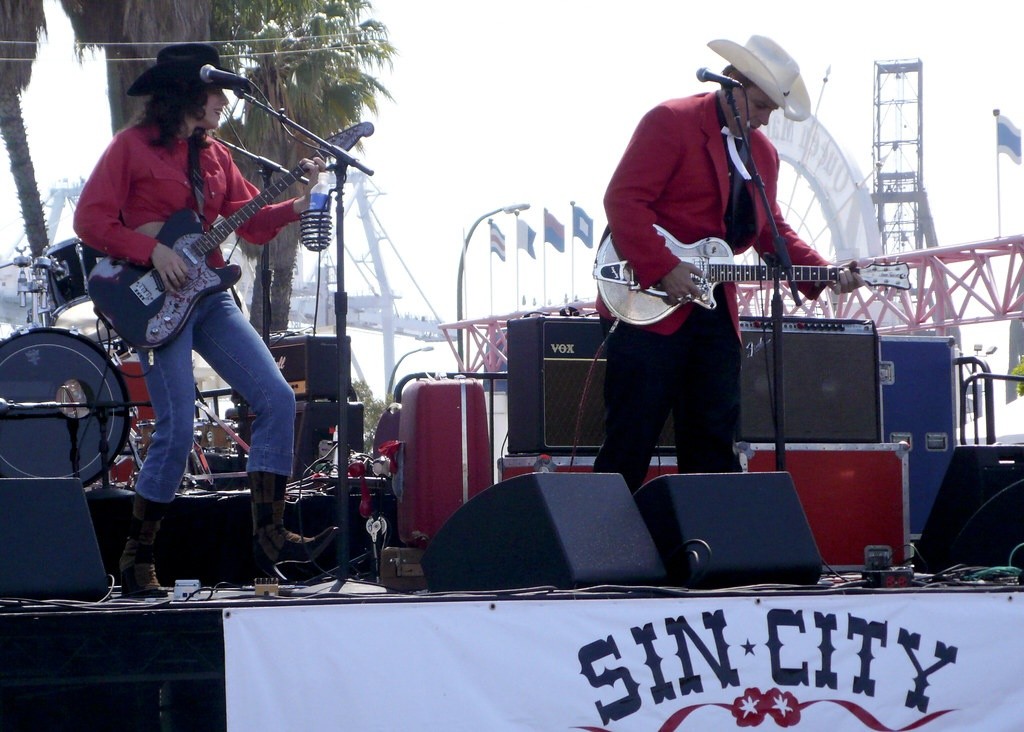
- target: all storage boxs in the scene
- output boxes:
[499,456,751,486]
[878,334,956,541]
[733,442,911,571]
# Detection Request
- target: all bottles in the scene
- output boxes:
[304,172,331,246]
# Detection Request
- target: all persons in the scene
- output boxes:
[592,35,865,495]
[75,41,326,598]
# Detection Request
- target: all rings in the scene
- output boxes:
[676,297,683,302]
[315,171,318,175]
[686,293,692,300]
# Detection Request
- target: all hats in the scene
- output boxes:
[705,33,813,122]
[126,42,254,98]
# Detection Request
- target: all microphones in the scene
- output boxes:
[696,68,743,88]
[200,64,251,87]
[0,398,9,410]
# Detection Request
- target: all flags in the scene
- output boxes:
[491,223,505,261]
[545,212,564,253]
[997,115,1022,164]
[517,218,536,259]
[573,206,593,249]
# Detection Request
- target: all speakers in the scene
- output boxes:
[240,402,365,490]
[419,472,668,594]
[0,476,110,609]
[737,315,882,444]
[632,471,822,591]
[507,315,680,458]
[914,445,1024,575]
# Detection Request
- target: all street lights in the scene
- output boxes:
[455,202,532,371]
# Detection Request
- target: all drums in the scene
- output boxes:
[41,235,125,349]
[0,326,132,488]
[136,417,236,460]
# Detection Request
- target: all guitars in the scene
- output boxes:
[592,222,912,335]
[87,121,375,350]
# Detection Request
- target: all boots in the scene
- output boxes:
[118,491,177,599]
[244,470,339,581]
